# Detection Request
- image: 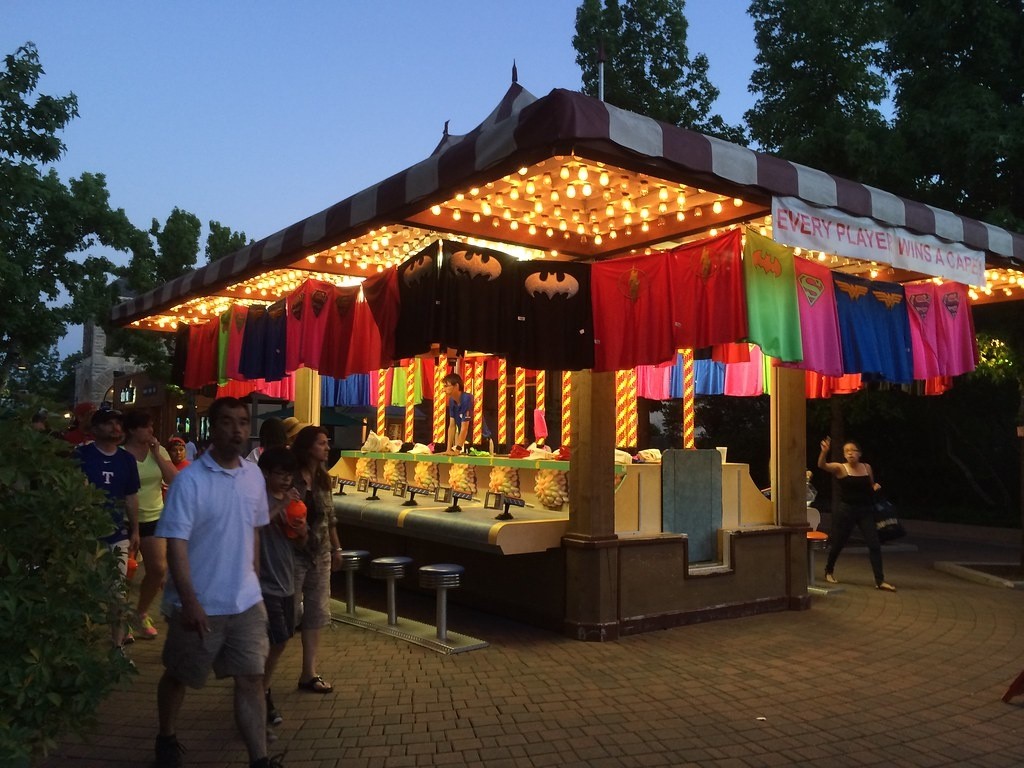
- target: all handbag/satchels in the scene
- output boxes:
[875,509,907,543]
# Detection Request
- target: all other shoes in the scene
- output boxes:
[877,581,896,591]
[825,571,837,583]
[156,734,188,768]
[250,753,286,768]
[265,692,283,726]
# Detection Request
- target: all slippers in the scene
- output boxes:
[298,675,333,693]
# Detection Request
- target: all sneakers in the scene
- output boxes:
[130,613,159,639]
[120,623,136,642]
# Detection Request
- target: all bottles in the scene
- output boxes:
[286,498,307,540]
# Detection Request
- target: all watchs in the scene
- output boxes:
[456,446,462,450]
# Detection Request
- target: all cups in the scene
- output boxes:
[716,447,728,464]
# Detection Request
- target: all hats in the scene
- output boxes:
[165,437,186,450]
[283,417,313,439]
[90,408,123,421]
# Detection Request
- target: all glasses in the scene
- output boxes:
[843,449,859,453]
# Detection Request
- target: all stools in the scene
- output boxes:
[806,532,828,587]
[419,564,464,640]
[372,556,413,626]
[340,550,370,613]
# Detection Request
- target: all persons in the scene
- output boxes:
[440,374,492,457]
[31,396,343,768]
[817,436,898,591]
